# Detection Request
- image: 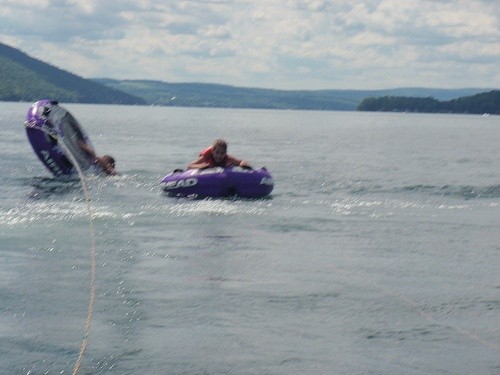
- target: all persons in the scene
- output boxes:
[188,140,248,170]
[79,141,116,175]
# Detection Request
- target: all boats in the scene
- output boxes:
[25,99,101,180]
[159,164,277,201]
